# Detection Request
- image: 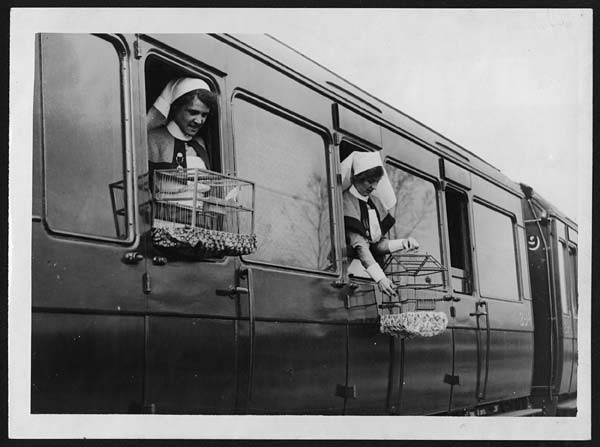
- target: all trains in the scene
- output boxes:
[31,32,578,415]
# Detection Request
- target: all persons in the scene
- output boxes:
[339,151,420,297]
[144,75,214,210]
